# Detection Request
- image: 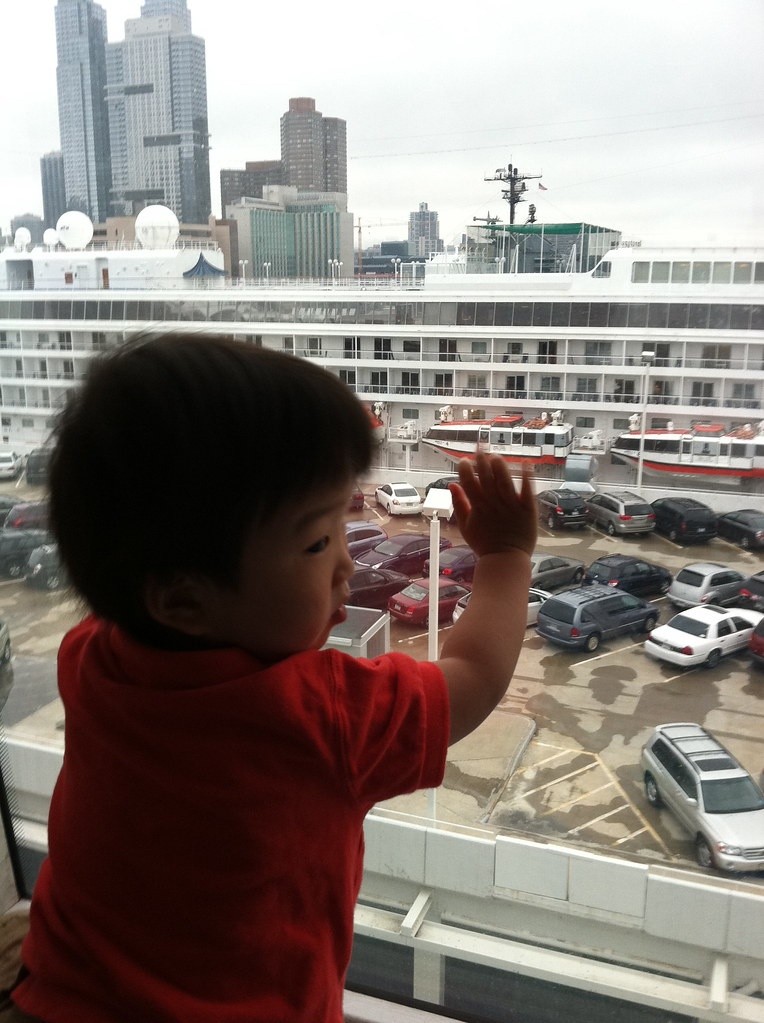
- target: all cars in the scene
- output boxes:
[650,497,718,544]
[388,576,473,630]
[665,561,748,608]
[0,449,68,664]
[748,617,764,665]
[737,571,764,613]
[453,587,554,627]
[713,508,764,550]
[644,604,764,669]
[528,554,585,590]
[342,477,478,582]
[342,565,413,609]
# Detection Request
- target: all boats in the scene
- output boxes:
[423,405,574,470]
[360,406,764,484]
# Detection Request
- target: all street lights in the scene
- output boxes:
[636,351,654,492]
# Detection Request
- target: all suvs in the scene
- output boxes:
[580,553,673,595]
[639,722,764,873]
[537,489,590,530]
[584,490,656,536]
[535,585,661,653]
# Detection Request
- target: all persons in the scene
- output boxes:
[8,329,538,1023]
